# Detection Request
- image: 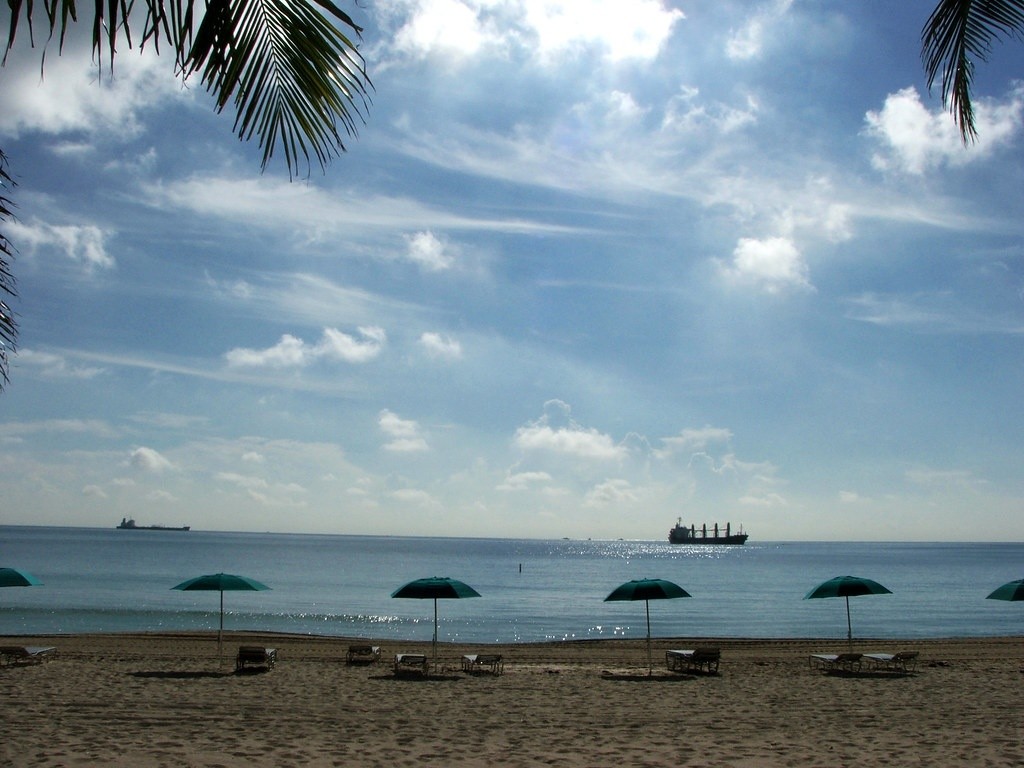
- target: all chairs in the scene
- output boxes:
[0,645,58,666]
[462,653,504,675]
[237,645,277,668]
[666,646,721,671]
[809,653,864,672]
[395,654,429,676]
[346,644,381,663]
[861,650,920,675]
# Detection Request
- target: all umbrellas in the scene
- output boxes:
[390,576,482,673]
[984,579,1024,601]
[168,572,273,669]
[802,575,893,671]
[603,577,693,679]
[0,566,44,587]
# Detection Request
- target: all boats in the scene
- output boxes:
[667,518,749,544]
[115,517,191,530]
